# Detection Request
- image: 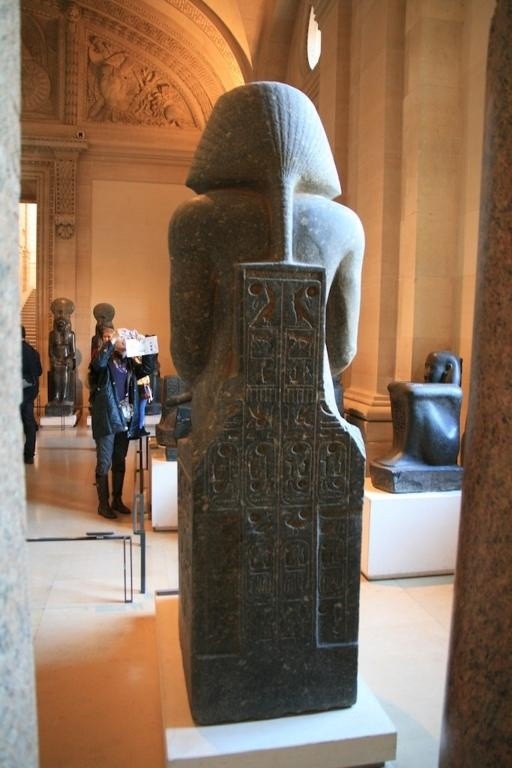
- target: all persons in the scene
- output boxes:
[20,327,42,464]
[167,80,365,430]
[91,328,160,440]
[373,351,462,468]
[89,35,169,122]
[49,318,76,400]
[88,329,154,519]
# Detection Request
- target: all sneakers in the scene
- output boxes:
[25,456,35,464]
[140,429,151,436]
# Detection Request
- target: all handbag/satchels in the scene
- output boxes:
[92,384,128,439]
[140,385,151,400]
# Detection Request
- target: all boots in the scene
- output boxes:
[96,473,131,519]
